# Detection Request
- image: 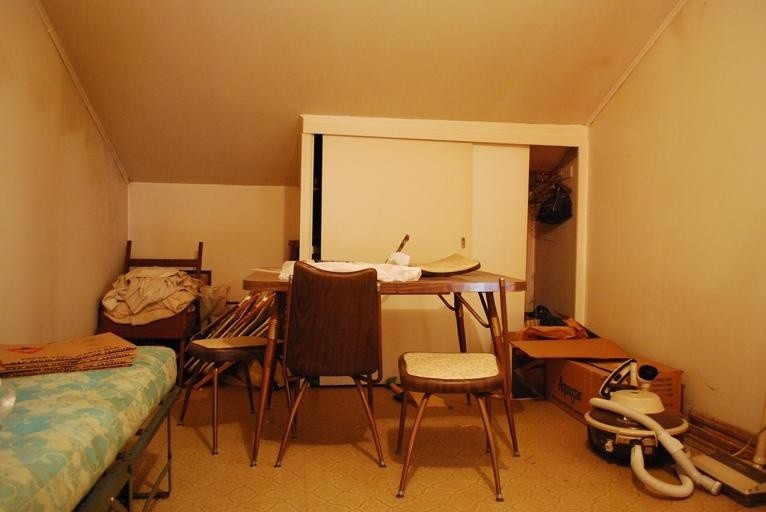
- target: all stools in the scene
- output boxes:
[394,351,505,502]
[178,335,301,455]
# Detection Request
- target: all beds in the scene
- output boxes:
[0,347,182,511]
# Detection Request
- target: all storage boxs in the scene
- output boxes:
[548,349,683,428]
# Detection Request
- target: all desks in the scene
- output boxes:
[243,266,527,467]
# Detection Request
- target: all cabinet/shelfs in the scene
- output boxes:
[299,115,588,387]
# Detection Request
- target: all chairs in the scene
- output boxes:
[274,260,384,467]
[99,240,203,388]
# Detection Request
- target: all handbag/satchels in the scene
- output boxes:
[534,190,573,228]
